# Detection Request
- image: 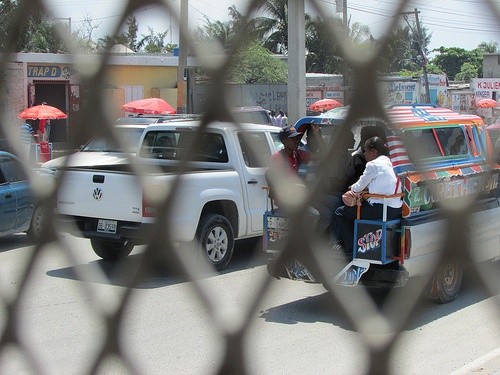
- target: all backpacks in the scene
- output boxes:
[266,148,301,214]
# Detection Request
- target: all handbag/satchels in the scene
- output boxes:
[342,191,367,207]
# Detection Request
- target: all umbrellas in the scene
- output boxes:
[16,104,68,140]
[307,98,343,112]
[122,98,176,115]
[478,98,497,108]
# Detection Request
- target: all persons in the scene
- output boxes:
[270,109,288,127]
[20,119,35,158]
[42,102,50,138]
[273,125,402,252]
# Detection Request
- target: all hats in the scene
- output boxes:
[279,126,303,138]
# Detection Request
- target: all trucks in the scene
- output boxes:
[262,106,500,305]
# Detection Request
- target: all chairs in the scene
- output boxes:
[262,186,289,217]
[354,192,405,225]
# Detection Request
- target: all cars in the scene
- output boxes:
[234,106,273,125]
[0,151,57,244]
[39,116,195,172]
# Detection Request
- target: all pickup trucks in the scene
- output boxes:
[54,118,310,273]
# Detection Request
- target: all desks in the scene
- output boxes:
[31,143,52,163]
